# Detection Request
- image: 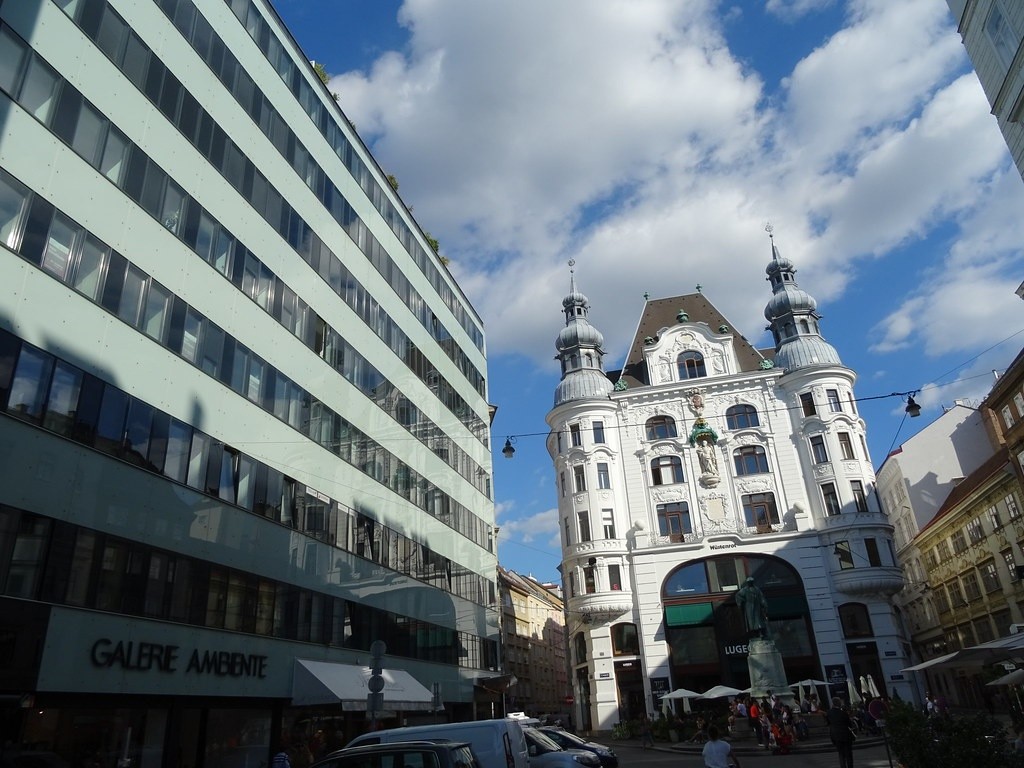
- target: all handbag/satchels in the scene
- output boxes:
[848,727,857,741]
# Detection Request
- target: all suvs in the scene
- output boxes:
[306,739,481,768]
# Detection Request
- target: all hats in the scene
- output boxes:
[832,697,841,707]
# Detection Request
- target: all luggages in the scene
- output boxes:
[795,715,809,741]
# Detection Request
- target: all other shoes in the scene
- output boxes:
[685,740,691,744]
[758,743,765,747]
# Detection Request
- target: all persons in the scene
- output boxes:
[685,689,820,753]
[641,713,654,750]
[696,441,717,474]
[702,725,740,768]
[924,695,952,720]
[272,728,343,768]
[1015,727,1024,768]
[827,697,855,768]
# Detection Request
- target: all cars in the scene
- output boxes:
[537,726,619,768]
[521,725,603,768]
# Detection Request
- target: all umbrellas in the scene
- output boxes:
[848,674,880,708]
[657,685,752,701]
[788,679,835,711]
[986,669,1024,685]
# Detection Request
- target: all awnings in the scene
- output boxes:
[291,658,445,711]
[900,631,1024,672]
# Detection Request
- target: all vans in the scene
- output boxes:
[346,719,530,768]
